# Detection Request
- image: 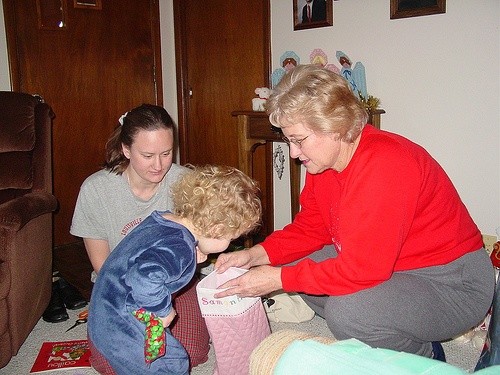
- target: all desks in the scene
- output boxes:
[231,109,386,247]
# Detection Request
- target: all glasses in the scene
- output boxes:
[282,133,315,148]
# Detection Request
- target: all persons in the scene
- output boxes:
[214,63,496,359]
[88,165,262,375]
[69,104,210,375]
[302,0,326,23]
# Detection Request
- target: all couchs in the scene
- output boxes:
[0,91,57,370]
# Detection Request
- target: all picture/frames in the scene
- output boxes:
[390,0,446,20]
[293,0,333,31]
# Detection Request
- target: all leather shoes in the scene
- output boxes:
[42,276,69,324]
[51,270,88,310]
